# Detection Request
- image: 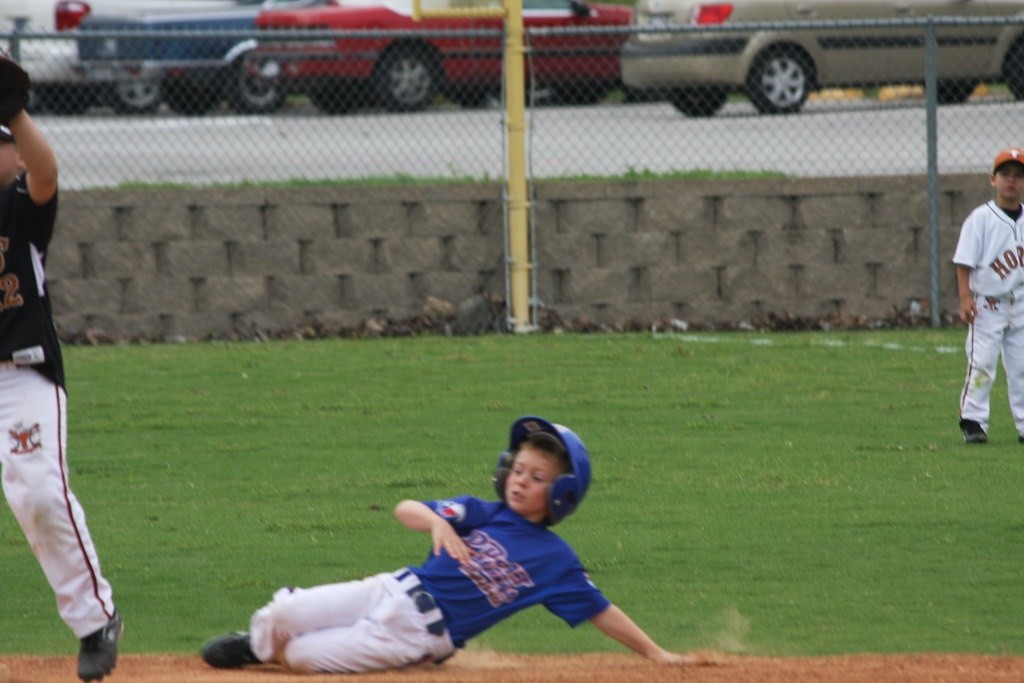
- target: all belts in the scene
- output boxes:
[397,571,453,664]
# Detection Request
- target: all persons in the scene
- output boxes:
[199,416,691,674]
[952,148,1024,444]
[0,49,125,683]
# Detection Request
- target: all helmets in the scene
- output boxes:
[492,417,591,526]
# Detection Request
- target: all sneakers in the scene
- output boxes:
[201,631,263,670]
[76,609,124,683]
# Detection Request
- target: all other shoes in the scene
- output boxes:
[959,419,987,443]
[1019,437,1024,443]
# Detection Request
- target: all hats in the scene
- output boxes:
[994,148,1024,174]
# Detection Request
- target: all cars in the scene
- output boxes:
[241,0,633,116]
[618,0,1024,119]
[0,1,334,116]
